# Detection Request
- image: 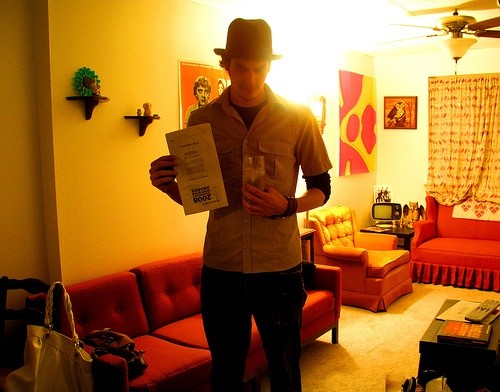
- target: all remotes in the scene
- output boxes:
[465,299,500,322]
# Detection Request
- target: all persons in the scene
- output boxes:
[217,80,225,97]
[148,17,332,392]
[185,75,211,128]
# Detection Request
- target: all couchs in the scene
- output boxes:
[304,206,413,313]
[25,252,341,392]
[409,195,500,292]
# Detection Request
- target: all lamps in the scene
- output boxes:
[442,33,478,83]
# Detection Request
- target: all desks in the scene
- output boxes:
[360,224,414,250]
[300,226,315,264]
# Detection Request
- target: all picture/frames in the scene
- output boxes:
[177,58,231,129]
[384,96,418,129]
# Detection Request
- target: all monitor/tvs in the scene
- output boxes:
[372,202,402,224]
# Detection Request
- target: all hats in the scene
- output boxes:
[214,18,284,60]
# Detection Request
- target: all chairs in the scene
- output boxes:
[0,276,63,387]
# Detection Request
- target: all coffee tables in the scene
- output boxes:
[418,298,500,392]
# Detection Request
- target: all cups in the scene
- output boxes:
[242,155,264,207]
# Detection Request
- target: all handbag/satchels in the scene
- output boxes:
[81,327,148,381]
[0,280,94,392]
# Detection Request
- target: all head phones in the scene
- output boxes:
[403,369,439,392]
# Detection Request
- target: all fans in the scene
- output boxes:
[400,8,500,39]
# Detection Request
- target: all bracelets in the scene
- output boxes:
[284,195,298,218]
